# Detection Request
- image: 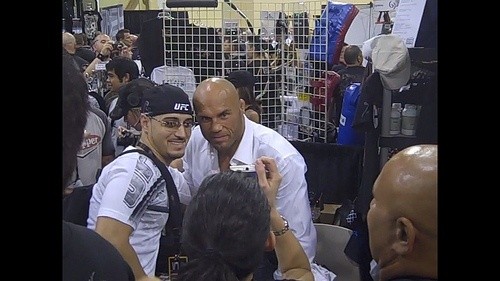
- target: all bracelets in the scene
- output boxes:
[97,53,105,61]
[272,215,289,237]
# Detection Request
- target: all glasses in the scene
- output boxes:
[148,116,195,131]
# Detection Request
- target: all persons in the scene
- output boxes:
[64,20,438,281]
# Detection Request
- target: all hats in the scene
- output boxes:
[108,77,158,120]
[140,83,194,116]
[371,34,411,91]
[227,70,257,88]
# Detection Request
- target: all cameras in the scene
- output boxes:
[230,165,265,180]
[111,42,123,51]
[121,126,132,138]
